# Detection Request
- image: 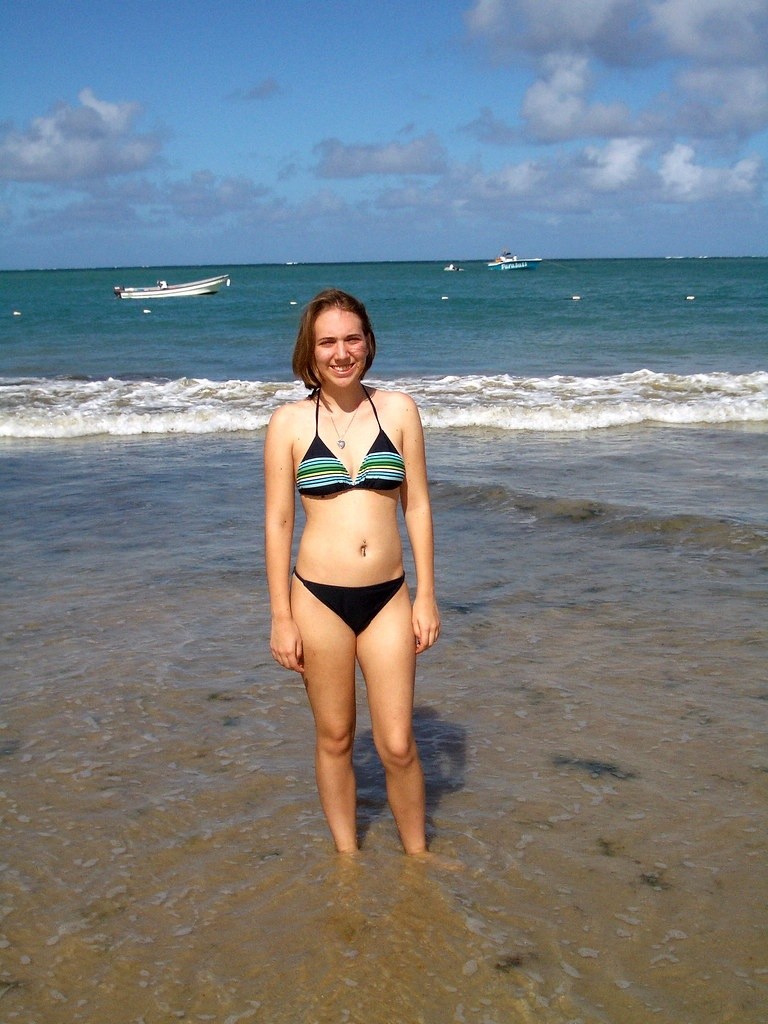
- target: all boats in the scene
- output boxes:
[487,247,543,274]
[113,273,230,300]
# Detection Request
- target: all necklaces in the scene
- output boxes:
[320,396,362,449]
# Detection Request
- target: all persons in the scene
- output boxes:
[263,287,442,855]
